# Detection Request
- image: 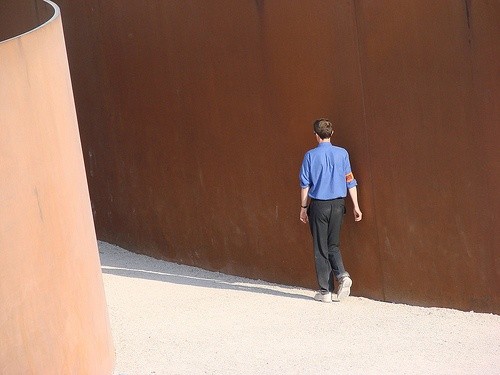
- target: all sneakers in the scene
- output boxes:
[314,292,332,302]
[337,277,353,302]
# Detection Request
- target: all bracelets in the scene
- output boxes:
[301,205,307,208]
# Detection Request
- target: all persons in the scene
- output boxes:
[299,117,363,302]
